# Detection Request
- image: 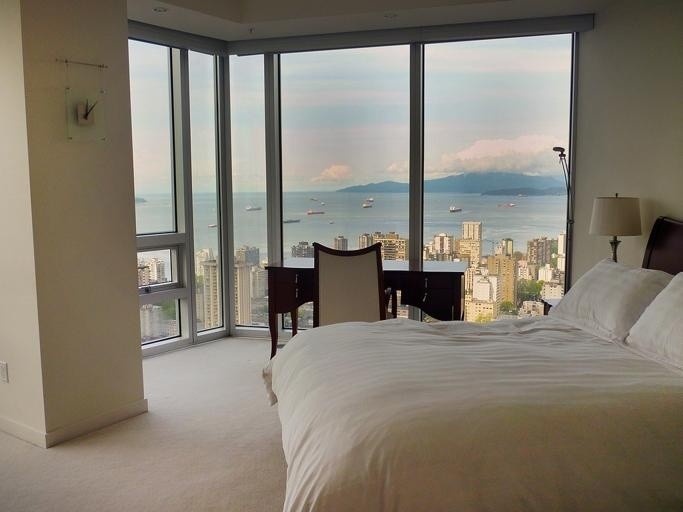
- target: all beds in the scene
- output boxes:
[262,216,683,511]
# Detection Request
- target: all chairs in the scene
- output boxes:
[312,242,397,327]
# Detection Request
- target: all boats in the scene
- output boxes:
[450,207,461,212]
[307,209,325,214]
[366,197,374,202]
[208,224,217,227]
[362,203,372,207]
[284,218,301,223]
[246,206,261,211]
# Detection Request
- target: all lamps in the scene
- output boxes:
[588,192,642,262]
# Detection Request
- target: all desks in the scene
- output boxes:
[265,256,469,360]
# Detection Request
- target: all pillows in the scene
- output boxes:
[620,271,683,370]
[547,253,675,345]
